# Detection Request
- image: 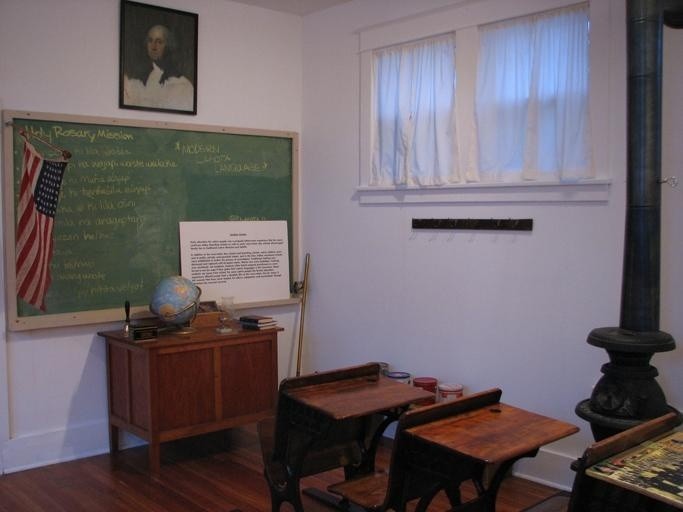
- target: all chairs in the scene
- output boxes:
[515,409,682,512]
[302,381,502,509]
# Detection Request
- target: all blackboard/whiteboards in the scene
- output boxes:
[1,110,302,331]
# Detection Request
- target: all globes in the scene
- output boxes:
[149,275,201,334]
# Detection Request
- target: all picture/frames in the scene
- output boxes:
[115,2,200,116]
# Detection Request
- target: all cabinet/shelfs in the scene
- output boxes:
[88,317,284,477]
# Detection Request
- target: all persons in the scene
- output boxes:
[592,452,683,498]
[119,19,195,112]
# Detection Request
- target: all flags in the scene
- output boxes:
[14,133,68,313]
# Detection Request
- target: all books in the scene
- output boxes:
[237,314,273,323]
[241,325,277,331]
[240,321,278,327]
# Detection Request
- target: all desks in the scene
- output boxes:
[265,358,437,509]
[402,395,580,512]
